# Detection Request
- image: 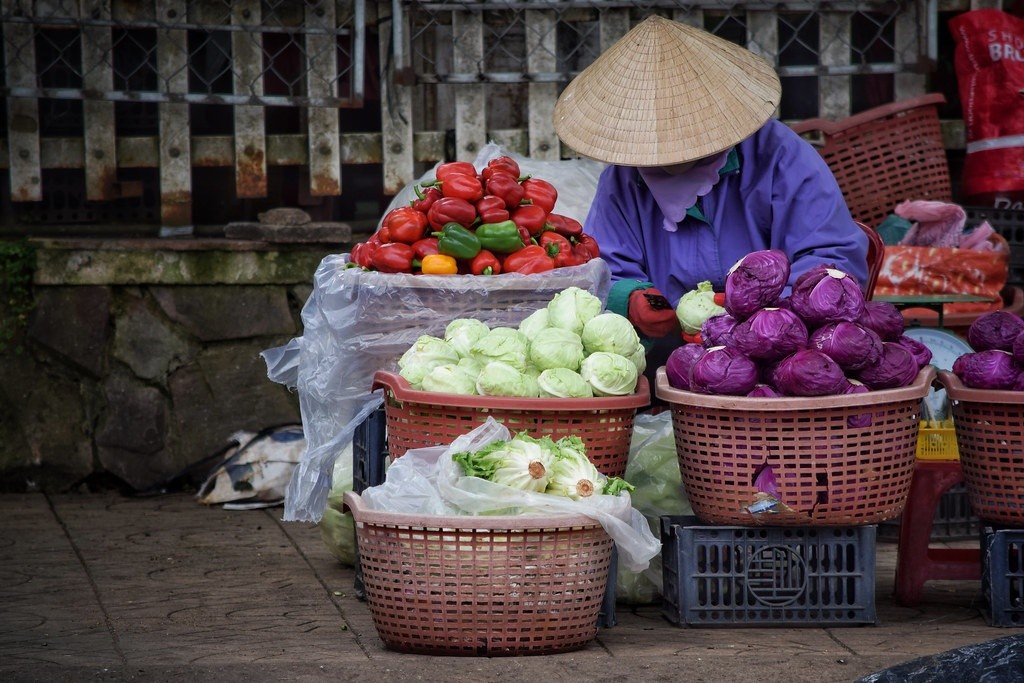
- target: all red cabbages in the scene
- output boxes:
[667,250,931,505]
[953,310,1024,496]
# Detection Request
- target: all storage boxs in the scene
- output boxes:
[977,526,1024,629]
[915,420,959,459]
[660,514,882,628]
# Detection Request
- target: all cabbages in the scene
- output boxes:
[676,281,728,334]
[397,287,646,499]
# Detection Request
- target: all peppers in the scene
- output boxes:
[349,155,599,275]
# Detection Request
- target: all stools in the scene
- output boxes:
[893,459,1017,609]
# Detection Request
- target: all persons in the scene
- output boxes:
[551,15,870,421]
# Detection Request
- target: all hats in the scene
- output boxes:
[554,15,782,167]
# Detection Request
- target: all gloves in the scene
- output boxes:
[630,289,673,340]
[681,294,723,343]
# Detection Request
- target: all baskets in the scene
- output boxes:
[933,370,1024,523]
[981,528,1024,628]
[952,205,1024,285]
[343,490,614,656]
[352,404,383,598]
[370,375,651,485]
[336,257,594,372]
[655,366,936,526]
[898,287,1024,341]
[916,420,959,460]
[660,515,881,628]
[789,93,950,227]
